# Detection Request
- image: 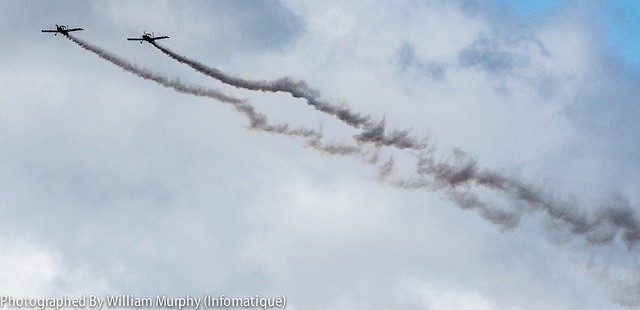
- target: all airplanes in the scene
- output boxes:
[41,24,85,36]
[127,32,170,44]
